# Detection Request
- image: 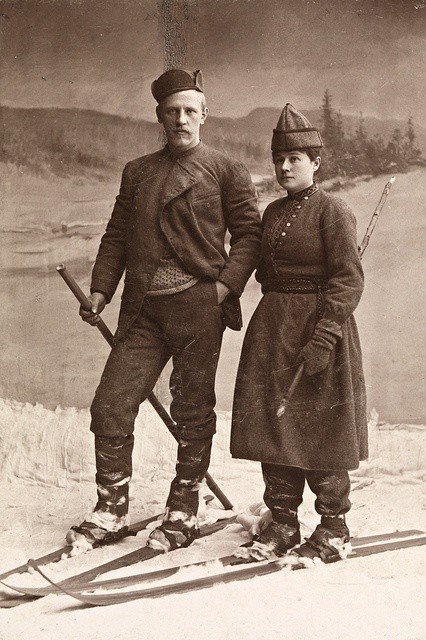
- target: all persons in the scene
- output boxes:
[230,104,369,563]
[67,68,262,552]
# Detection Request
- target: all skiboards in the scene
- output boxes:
[0,495,250,606]
[2,530,426,604]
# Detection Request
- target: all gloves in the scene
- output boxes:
[295,329,337,377]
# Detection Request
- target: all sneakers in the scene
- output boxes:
[66,517,129,548]
[146,513,200,551]
[290,524,350,561]
[239,521,300,562]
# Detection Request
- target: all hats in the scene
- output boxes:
[150,69,204,102]
[271,103,324,151]
